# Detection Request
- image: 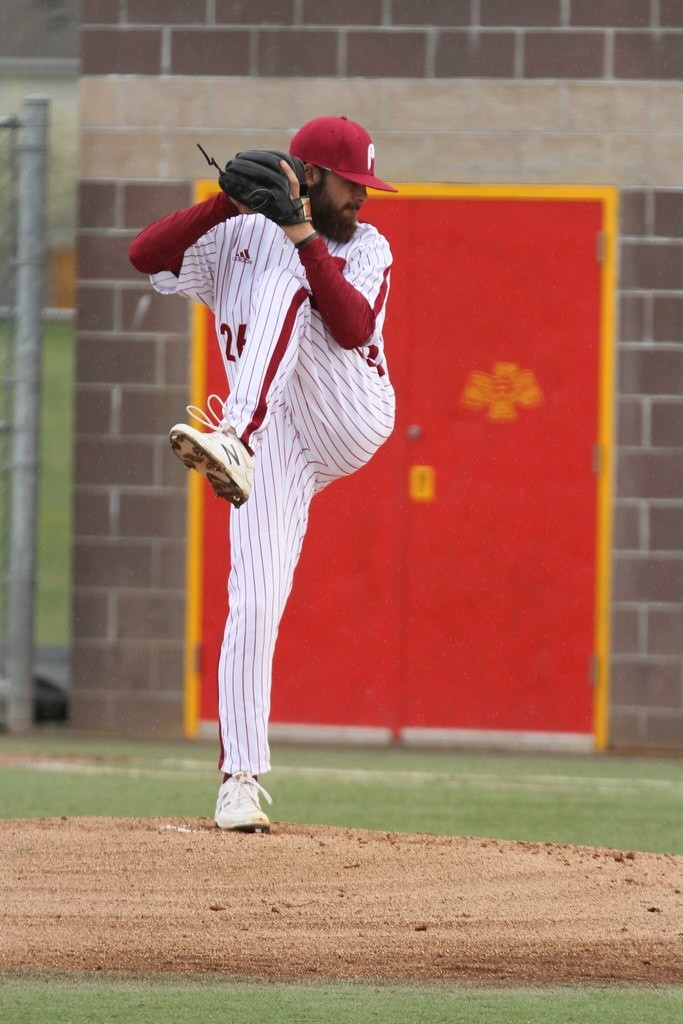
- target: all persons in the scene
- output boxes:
[128,118,398,836]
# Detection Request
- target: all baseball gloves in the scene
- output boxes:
[196,141,311,225]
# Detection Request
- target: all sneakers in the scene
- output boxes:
[170,422,256,506]
[217,777,272,831]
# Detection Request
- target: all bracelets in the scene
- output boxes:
[293,229,319,249]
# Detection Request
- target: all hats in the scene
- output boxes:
[289,116,398,195]
